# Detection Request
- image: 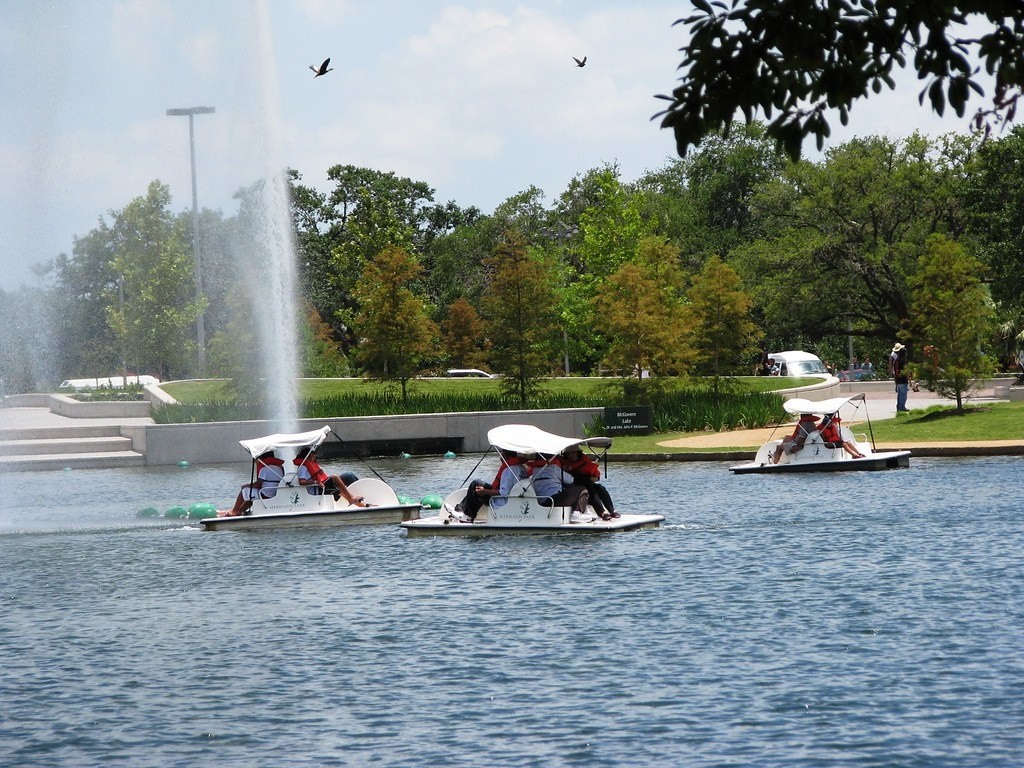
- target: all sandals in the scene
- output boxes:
[611,511,621,518]
[602,514,612,520]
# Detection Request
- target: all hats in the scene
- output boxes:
[300,447,316,457]
[892,343,905,351]
[562,444,583,455]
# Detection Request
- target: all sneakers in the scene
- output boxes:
[452,511,471,523]
[444,503,455,514]
[570,513,592,523]
[584,510,597,520]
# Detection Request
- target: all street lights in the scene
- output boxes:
[164,104,216,380]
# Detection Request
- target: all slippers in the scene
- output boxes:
[348,496,364,507]
[223,511,236,516]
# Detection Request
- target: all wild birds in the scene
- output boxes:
[572,57,587,67]
[308,57,334,79]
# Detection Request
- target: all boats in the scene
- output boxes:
[197,424,424,533]
[400,423,666,537]
[728,392,912,475]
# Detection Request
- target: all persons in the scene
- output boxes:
[823,356,875,381]
[765,358,779,376]
[817,413,866,459]
[923,338,939,393]
[293,448,364,504]
[223,451,285,517]
[444,449,529,523]
[769,414,817,464]
[890,343,912,412]
[532,444,621,523]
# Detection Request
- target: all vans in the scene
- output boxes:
[766,350,831,377]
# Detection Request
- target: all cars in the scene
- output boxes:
[837,368,878,381]
[446,368,492,379]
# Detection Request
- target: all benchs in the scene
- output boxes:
[486,475,601,524]
[788,425,870,464]
[248,471,336,514]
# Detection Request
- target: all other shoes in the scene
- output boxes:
[860,454,866,457]
[852,454,863,459]
[897,408,910,412]
